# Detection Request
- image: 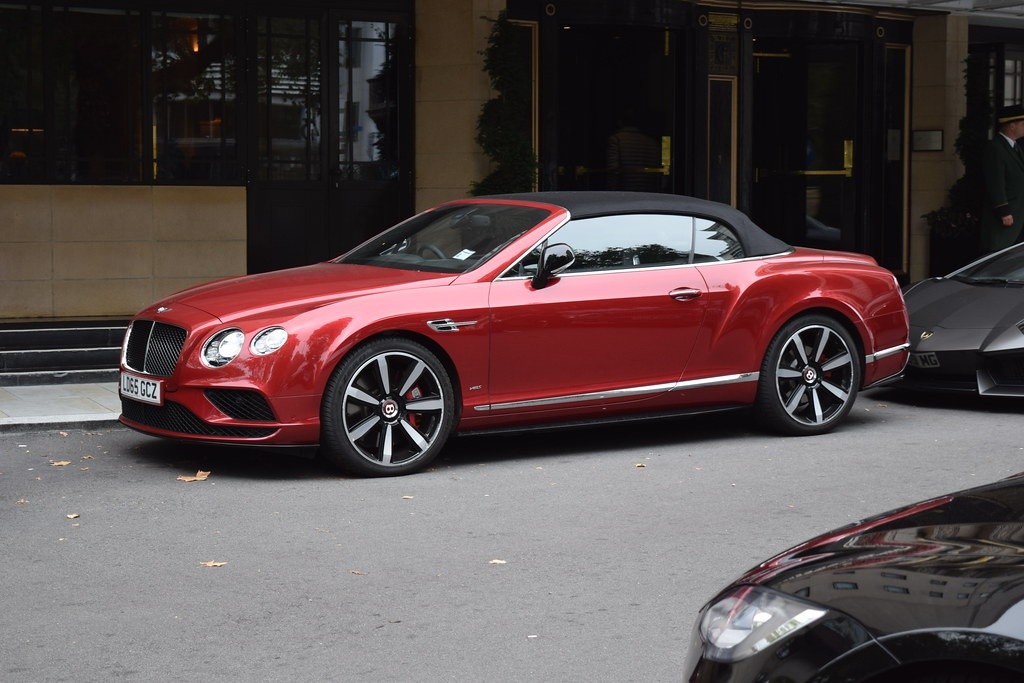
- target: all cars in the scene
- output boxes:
[678,471,1024,683]
[117,190,910,477]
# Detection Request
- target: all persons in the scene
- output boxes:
[980,106,1024,257]
[607,105,669,193]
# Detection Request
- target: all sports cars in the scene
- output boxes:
[888,240,1023,398]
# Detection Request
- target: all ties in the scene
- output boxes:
[1013,142,1020,154]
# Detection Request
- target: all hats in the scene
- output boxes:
[997,105,1024,123]
